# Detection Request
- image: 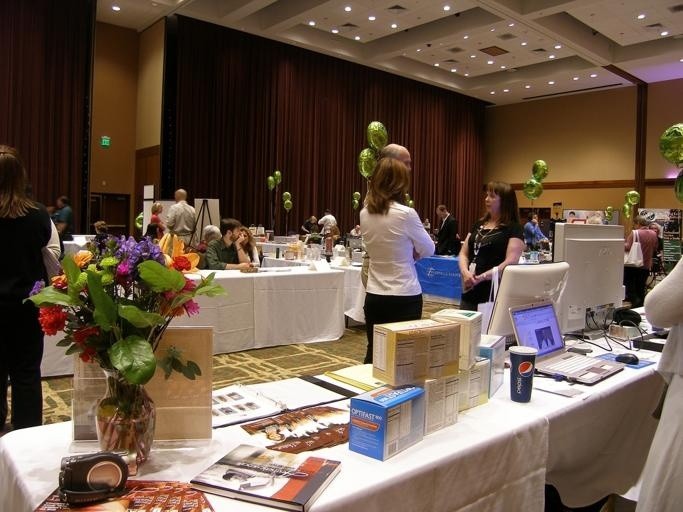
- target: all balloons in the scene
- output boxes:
[673,169,683,204]
[531,159,548,182]
[282,191,291,202]
[283,199,292,211]
[521,179,543,203]
[272,170,282,185]
[657,122,683,167]
[266,176,275,191]
[350,121,415,211]
[624,191,640,207]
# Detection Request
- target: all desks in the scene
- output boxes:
[0,307,672,512]
[413,234,672,307]
[2,234,366,380]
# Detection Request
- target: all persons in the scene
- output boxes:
[86,219,111,258]
[142,183,259,274]
[317,208,337,237]
[348,225,362,238]
[634,255,682,511]
[362,159,437,365]
[455,177,526,314]
[297,214,317,236]
[420,202,665,311]
[360,143,414,290]
[49,194,74,261]
[0,144,61,431]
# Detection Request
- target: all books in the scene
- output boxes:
[30,474,212,512]
[192,440,343,512]
[212,358,386,452]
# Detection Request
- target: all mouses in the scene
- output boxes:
[616,353,638,365]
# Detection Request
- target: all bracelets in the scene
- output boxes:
[482,270,486,281]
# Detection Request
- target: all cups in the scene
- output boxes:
[283,243,321,261]
[530,251,538,262]
[507,344,538,403]
[524,253,529,262]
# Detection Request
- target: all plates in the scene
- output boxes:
[352,263,362,267]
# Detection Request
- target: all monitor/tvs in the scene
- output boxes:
[552,222,626,333]
[486,261,570,345]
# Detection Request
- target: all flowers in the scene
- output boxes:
[21,232,228,387]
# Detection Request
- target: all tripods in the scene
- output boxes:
[188,200,213,246]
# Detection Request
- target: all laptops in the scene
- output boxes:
[508,300,623,385]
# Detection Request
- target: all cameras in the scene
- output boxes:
[59,452,129,503]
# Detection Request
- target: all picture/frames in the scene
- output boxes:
[561,209,620,225]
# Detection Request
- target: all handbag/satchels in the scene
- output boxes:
[622,230,643,268]
[477,266,499,334]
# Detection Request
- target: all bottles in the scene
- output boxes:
[275,248,279,258]
[345,241,352,261]
[543,251,548,259]
[324,225,332,262]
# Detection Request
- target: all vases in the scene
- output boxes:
[92,365,157,468]
